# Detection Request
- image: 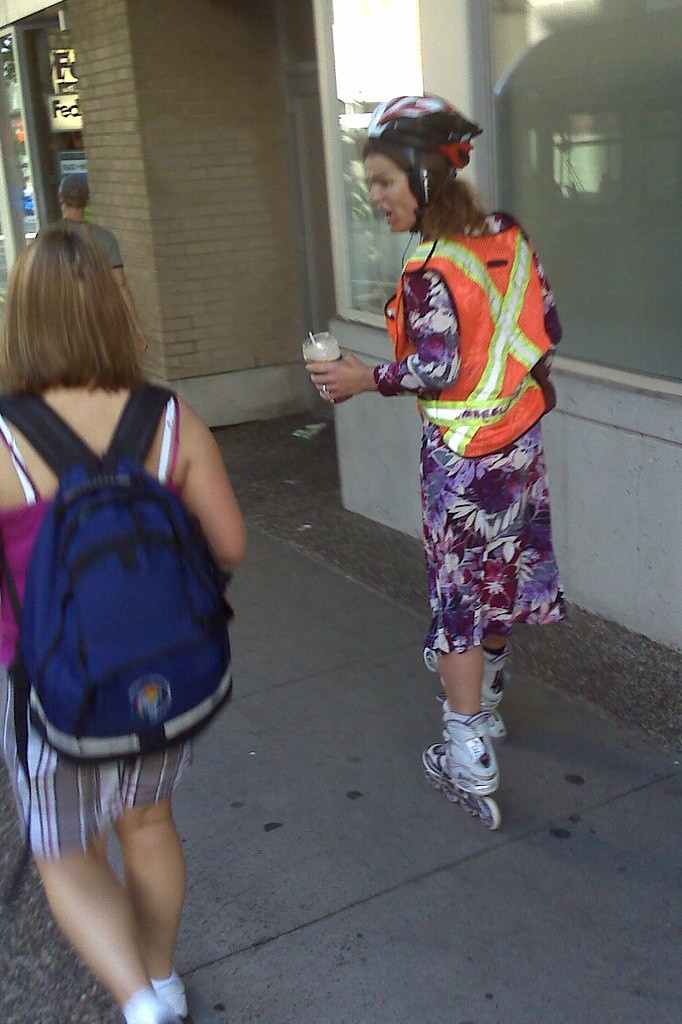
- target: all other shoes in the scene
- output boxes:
[150,969,188,1018]
[121,987,183,1023]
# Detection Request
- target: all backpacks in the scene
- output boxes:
[1,384,234,766]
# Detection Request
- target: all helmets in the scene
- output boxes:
[366,91,486,169]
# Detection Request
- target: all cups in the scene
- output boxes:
[302,331,354,404]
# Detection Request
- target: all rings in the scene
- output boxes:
[321,383,330,399]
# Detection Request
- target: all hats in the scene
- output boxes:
[58,173,90,196]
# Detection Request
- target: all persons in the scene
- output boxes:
[0,224,243,1024]
[308,96,569,829]
[37,172,146,354]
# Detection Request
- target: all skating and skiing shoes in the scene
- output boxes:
[422,700,502,831]
[422,646,511,743]
